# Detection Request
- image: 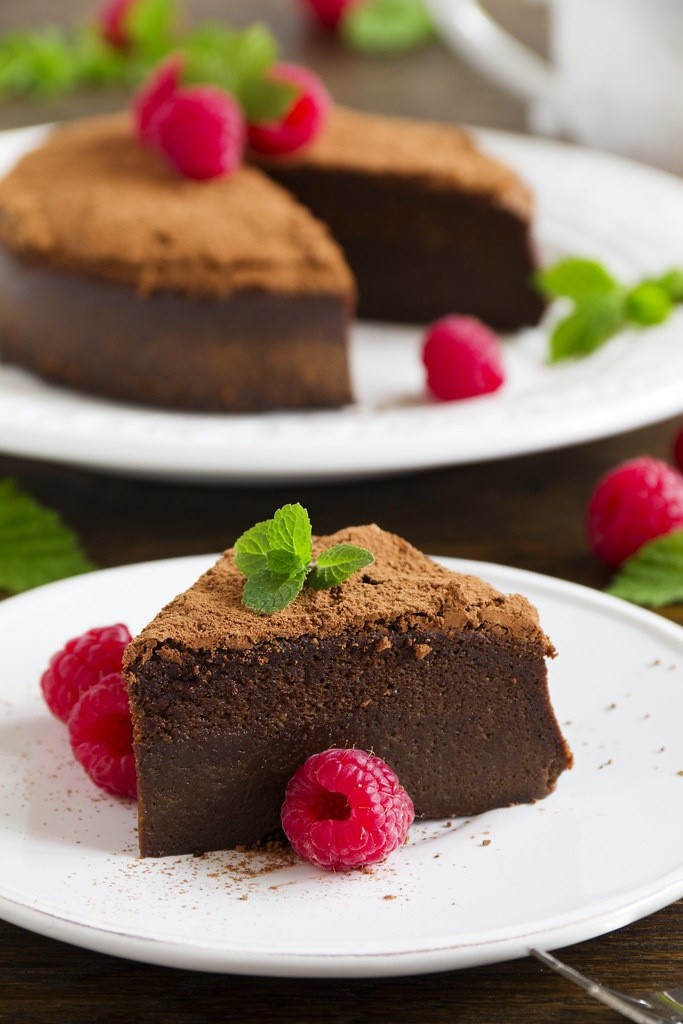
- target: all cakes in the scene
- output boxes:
[117,501,571,863]
[0,28,548,412]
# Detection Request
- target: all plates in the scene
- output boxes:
[1,552,682,979]
[1,122,683,485]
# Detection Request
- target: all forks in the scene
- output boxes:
[529,948,683,1024]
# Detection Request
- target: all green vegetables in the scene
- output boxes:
[600,527,683,605]
[533,255,682,360]
[0,0,438,98]
